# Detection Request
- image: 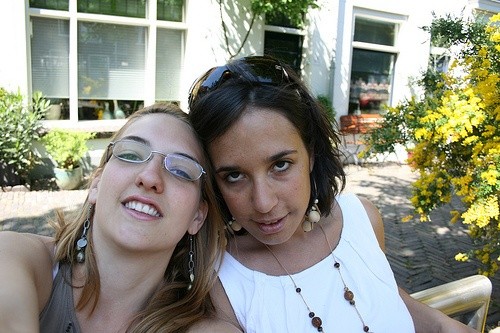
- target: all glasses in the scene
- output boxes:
[187,56,302,110]
[106,140,208,182]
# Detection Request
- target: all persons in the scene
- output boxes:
[0,102,242,333]
[185,55,485,333]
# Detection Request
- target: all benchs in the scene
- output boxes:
[340,113,404,165]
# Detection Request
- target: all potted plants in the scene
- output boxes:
[38,128,98,190]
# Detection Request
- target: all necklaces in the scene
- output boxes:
[268,226,370,333]
